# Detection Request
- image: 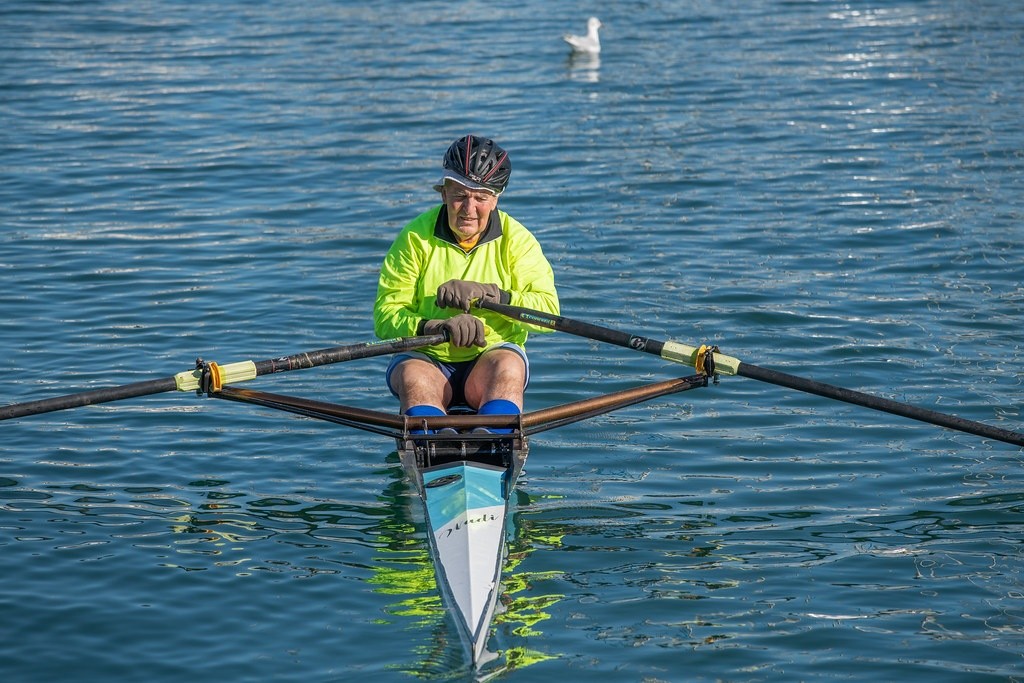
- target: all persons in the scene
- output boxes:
[373,135,560,448]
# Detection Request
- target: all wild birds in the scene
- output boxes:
[562,17,602,53]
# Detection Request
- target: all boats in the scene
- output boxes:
[406,419,520,672]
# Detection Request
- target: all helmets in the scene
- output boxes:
[432,135,511,197]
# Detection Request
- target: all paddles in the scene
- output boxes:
[0,329,448,418]
[475,297,1024,448]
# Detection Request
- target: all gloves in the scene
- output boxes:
[424,314,487,348]
[434,279,500,311]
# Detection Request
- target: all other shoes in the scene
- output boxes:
[436,428,458,435]
[472,428,491,434]
